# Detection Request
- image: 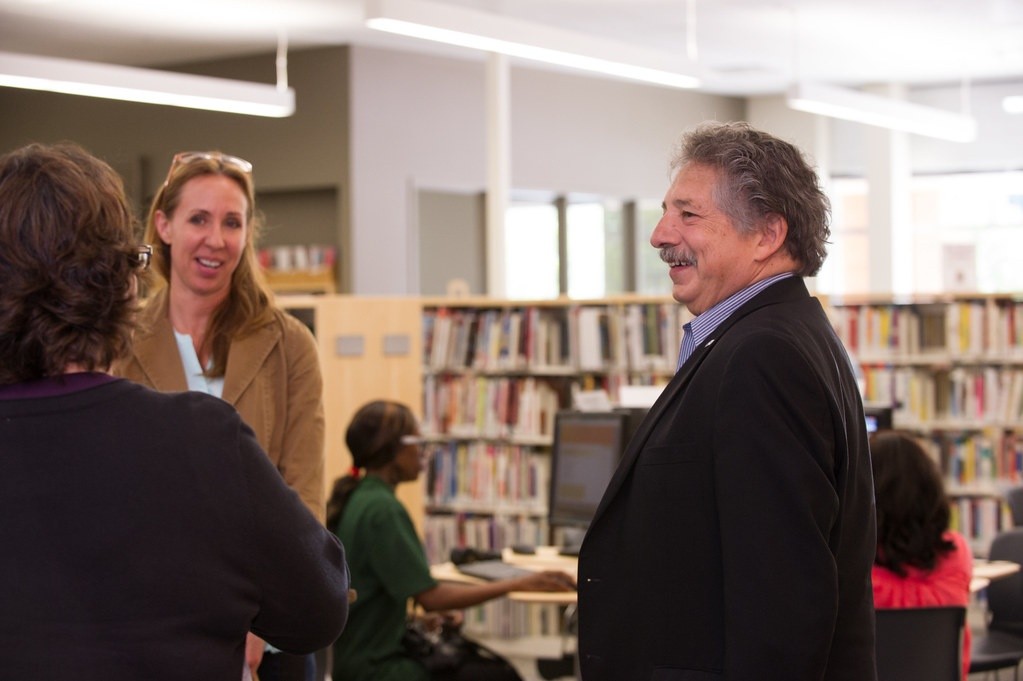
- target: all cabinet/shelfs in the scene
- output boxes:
[424,296,1023,681]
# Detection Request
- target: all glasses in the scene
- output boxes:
[400,436,426,454]
[136,245,153,268]
[164,150,253,186]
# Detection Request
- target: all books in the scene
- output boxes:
[421,307,694,637]
[819,302,1022,538]
[256,243,333,271]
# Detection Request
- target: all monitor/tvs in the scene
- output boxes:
[548,408,631,530]
[863,402,895,433]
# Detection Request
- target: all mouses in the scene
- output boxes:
[512,544,534,554]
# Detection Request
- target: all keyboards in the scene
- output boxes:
[458,559,530,582]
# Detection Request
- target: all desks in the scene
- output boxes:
[968,557,1019,592]
[428,544,580,680]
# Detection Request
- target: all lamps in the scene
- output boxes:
[786,74,976,146]
[0,23,297,119]
[365,0,700,88]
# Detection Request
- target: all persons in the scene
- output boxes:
[105,152,327,681]
[326,399,576,681]
[870,432,973,681]
[0,141,349,681]
[577,123,877,681]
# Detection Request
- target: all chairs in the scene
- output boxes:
[969,528,1022,681]
[872,603,968,681]
